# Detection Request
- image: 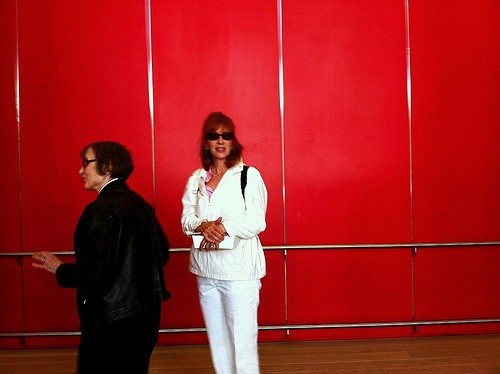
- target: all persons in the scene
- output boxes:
[182,112,267,373]
[30,141,169,374]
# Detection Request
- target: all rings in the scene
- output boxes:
[40,256,46,262]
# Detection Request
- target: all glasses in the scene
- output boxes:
[207,132,234,141]
[82,157,98,168]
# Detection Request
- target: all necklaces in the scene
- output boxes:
[210,166,223,179]
